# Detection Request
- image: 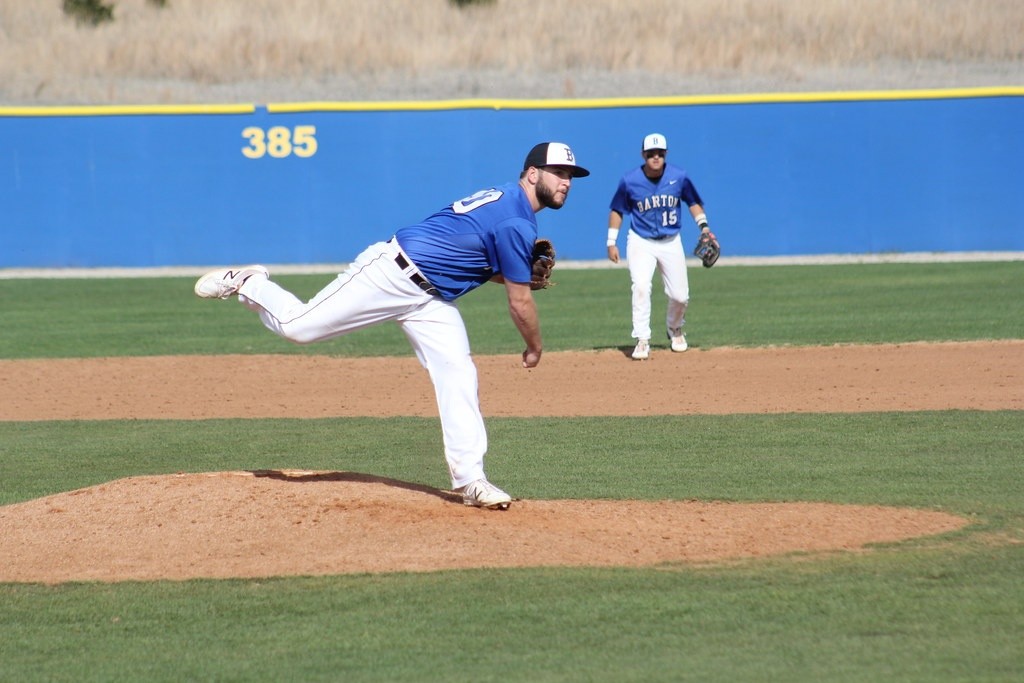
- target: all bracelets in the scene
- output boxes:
[699,223,708,232]
[606,240,615,247]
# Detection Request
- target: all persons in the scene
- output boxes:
[193,142,591,508]
[606,133,720,360]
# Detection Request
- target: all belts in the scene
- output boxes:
[387,239,441,297]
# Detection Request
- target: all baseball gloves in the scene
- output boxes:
[694,232,721,270]
[528,238,556,290]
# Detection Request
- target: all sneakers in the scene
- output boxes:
[631,339,650,361]
[462,479,512,509]
[666,327,688,353]
[194,265,269,299]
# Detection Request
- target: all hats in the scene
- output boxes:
[641,133,668,152]
[523,143,590,177]
